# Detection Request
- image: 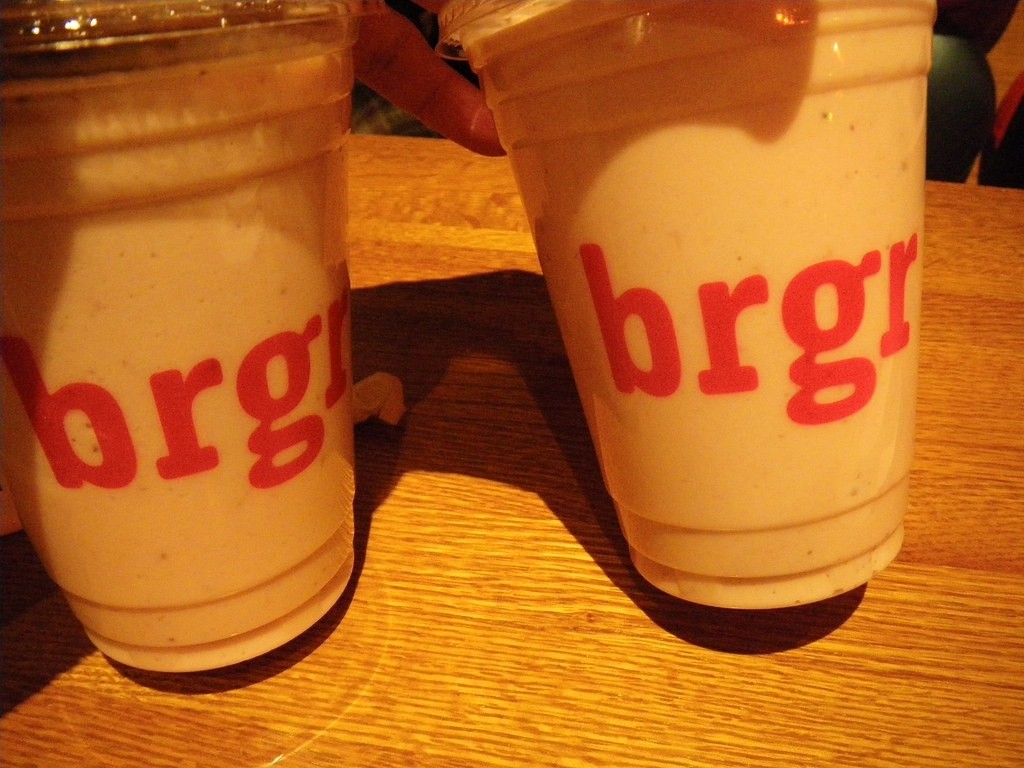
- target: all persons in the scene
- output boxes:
[351,0,509,158]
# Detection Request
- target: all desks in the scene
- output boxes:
[1,134,1024,768]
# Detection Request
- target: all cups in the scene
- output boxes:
[436,1,936,610]
[0,0,356,672]
